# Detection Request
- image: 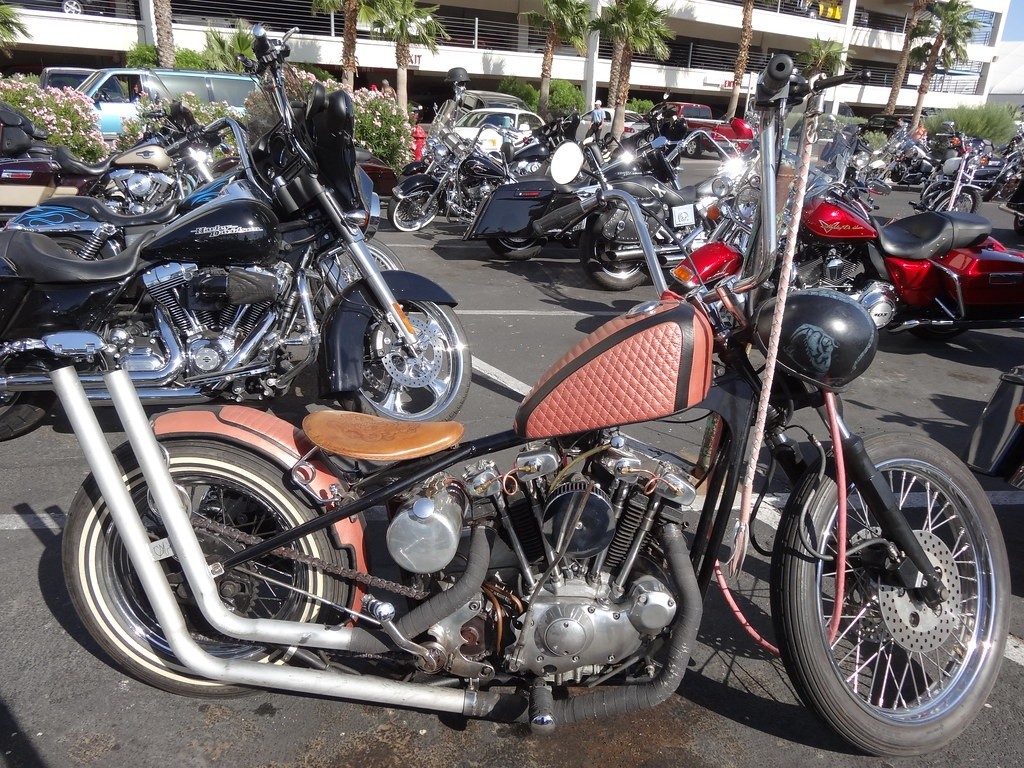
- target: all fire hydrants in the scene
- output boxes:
[411,126,428,162]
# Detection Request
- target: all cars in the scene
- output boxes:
[74,70,260,141]
[843,114,925,140]
[406,100,423,124]
[40,67,124,100]
[453,108,547,153]
[0,0,105,16]
[447,90,534,124]
[576,107,650,143]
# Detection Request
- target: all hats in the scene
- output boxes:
[382,79,389,87]
[594,100,602,106]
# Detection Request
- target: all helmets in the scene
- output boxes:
[444,67,471,82]
[751,289,879,392]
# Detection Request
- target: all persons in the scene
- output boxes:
[382,79,397,101]
[887,117,903,140]
[585,100,606,145]
[129,81,141,103]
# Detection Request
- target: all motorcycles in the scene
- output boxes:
[0,25,472,443]
[386,98,1023,495]
[50,54,1011,755]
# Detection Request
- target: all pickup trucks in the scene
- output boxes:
[646,101,737,158]
